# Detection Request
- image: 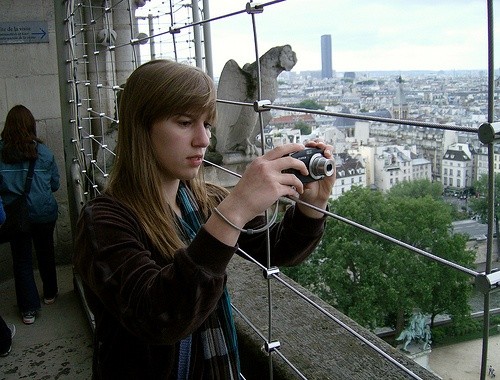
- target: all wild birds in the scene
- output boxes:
[211,44,297,157]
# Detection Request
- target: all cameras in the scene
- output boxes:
[281,146,335,183]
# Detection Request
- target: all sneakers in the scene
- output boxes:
[44,289,59,305]
[22,310,36,323]
[0,321,15,356]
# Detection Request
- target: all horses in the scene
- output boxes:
[395,315,432,351]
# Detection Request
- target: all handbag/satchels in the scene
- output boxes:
[3,192,37,232]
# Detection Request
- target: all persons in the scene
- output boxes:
[0,104,60,324]
[72,59,336,380]
[488,365,496,379]
